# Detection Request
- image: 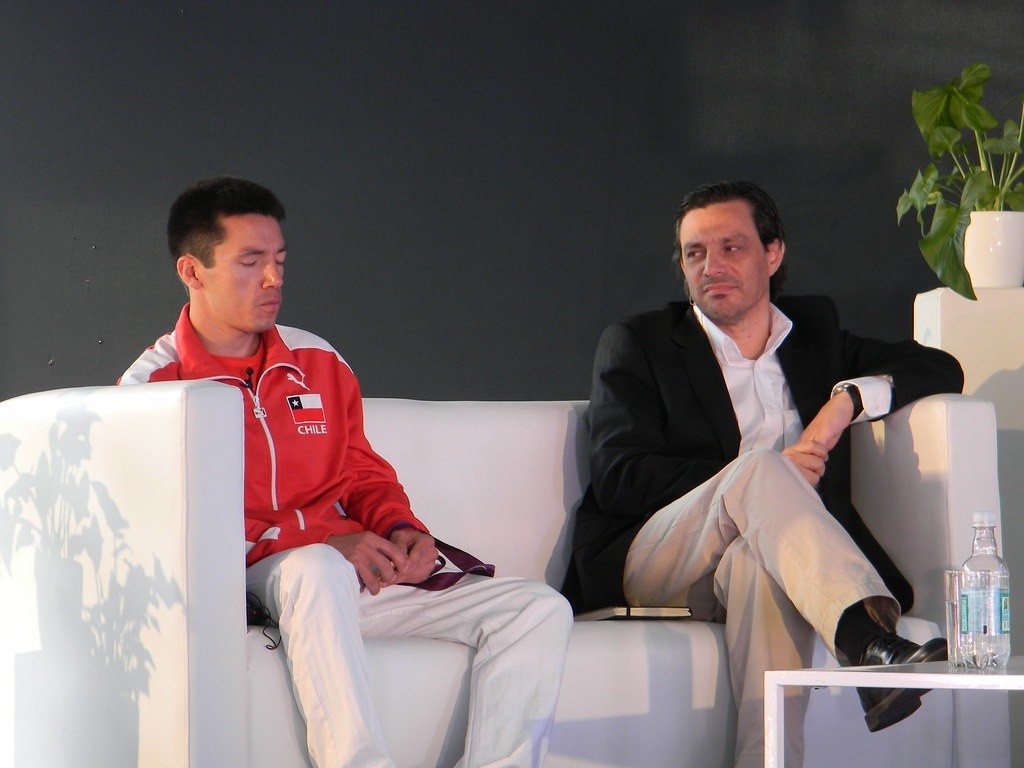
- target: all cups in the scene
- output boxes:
[943,569,992,667]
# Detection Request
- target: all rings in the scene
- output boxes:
[434,559,442,566]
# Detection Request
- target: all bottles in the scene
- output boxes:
[959,512,1009,669]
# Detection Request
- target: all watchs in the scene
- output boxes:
[831,382,864,423]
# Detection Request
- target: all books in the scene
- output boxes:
[572,605,692,620]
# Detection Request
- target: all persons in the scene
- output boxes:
[116,173,573,768]
[559,177,966,767]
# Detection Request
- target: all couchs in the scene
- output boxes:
[1,381,1010,767]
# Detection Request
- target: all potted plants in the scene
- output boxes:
[894,59,1023,301]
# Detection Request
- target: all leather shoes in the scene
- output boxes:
[855,633,951,734]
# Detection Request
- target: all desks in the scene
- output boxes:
[764,655,1015,768]
